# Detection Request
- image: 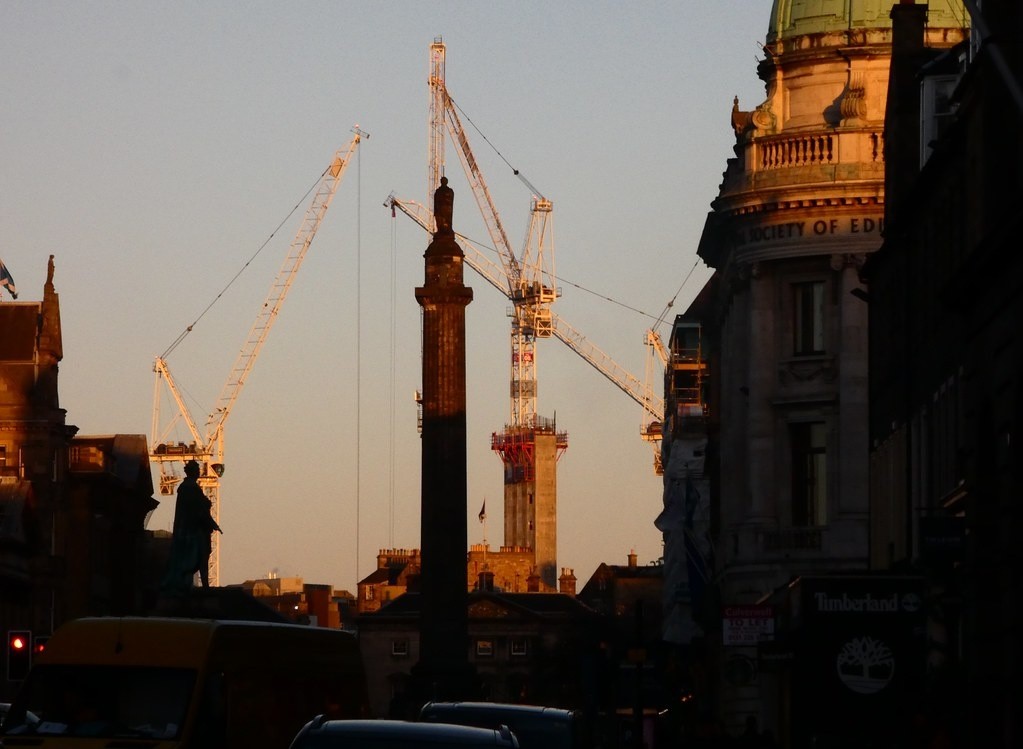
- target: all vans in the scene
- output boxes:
[0,612,389,749]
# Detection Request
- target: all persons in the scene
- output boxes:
[733,96,739,112]
[434,177,454,232]
[173,459,219,588]
[714,716,776,749]
[47,254,55,282]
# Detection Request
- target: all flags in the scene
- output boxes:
[479,499,486,524]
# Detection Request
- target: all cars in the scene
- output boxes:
[282,714,525,749]
[0,702,43,737]
[415,700,591,749]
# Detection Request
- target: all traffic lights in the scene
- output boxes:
[7,630,32,682]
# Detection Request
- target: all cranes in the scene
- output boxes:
[376,35,711,467]
[139,125,374,587]
